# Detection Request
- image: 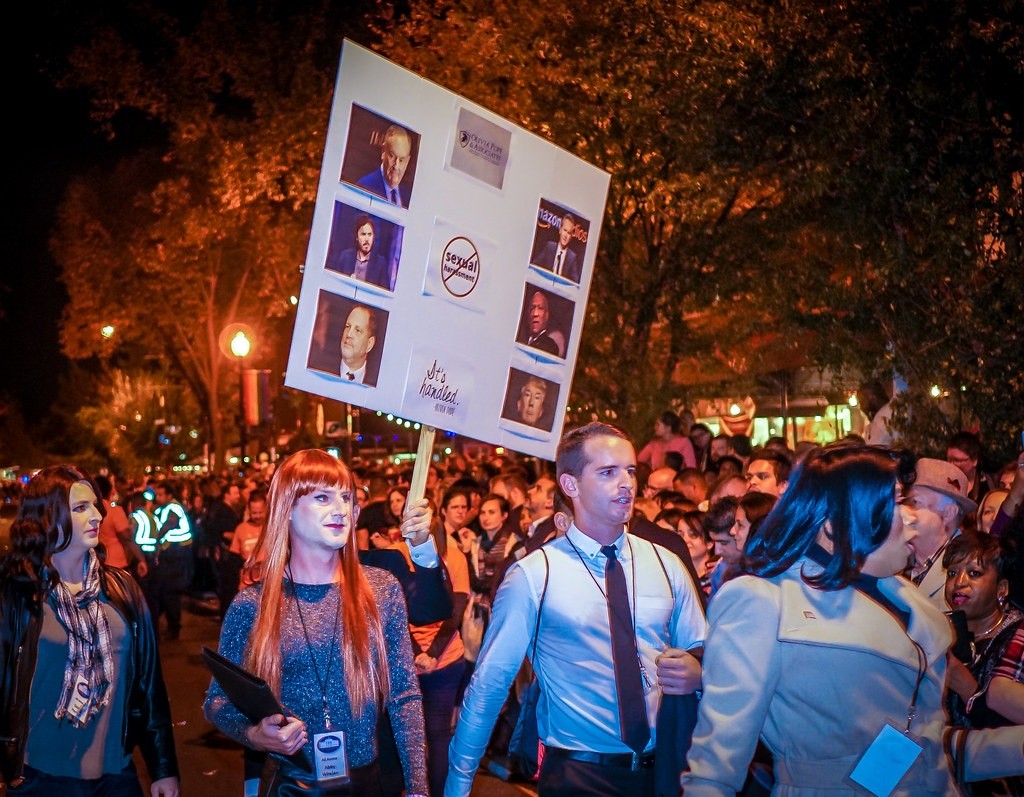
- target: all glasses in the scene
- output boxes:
[645,483,674,495]
[744,470,779,481]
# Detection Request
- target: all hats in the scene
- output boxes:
[913,459,979,514]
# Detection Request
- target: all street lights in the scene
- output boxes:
[229,332,251,523]
[835,385,858,441]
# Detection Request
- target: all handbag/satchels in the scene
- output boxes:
[461,595,484,662]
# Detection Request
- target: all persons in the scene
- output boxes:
[335,213,390,290]
[0,464,181,797]
[355,125,411,210]
[446,423,710,797]
[203,447,429,796]
[336,303,379,387]
[680,442,959,797]
[528,292,559,354]
[517,376,547,426]
[95,413,1024,797]
[532,214,580,284]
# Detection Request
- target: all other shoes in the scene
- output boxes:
[161,628,178,641]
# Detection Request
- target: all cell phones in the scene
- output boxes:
[941,609,973,663]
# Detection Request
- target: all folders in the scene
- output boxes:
[200,645,313,774]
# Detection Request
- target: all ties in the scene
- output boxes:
[390,189,397,204]
[347,373,354,383]
[600,544,651,756]
[556,251,562,275]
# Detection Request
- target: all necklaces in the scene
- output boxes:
[974,614,1004,639]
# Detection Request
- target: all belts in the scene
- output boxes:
[549,745,654,771]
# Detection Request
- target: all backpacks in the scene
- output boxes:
[505,537,674,780]
